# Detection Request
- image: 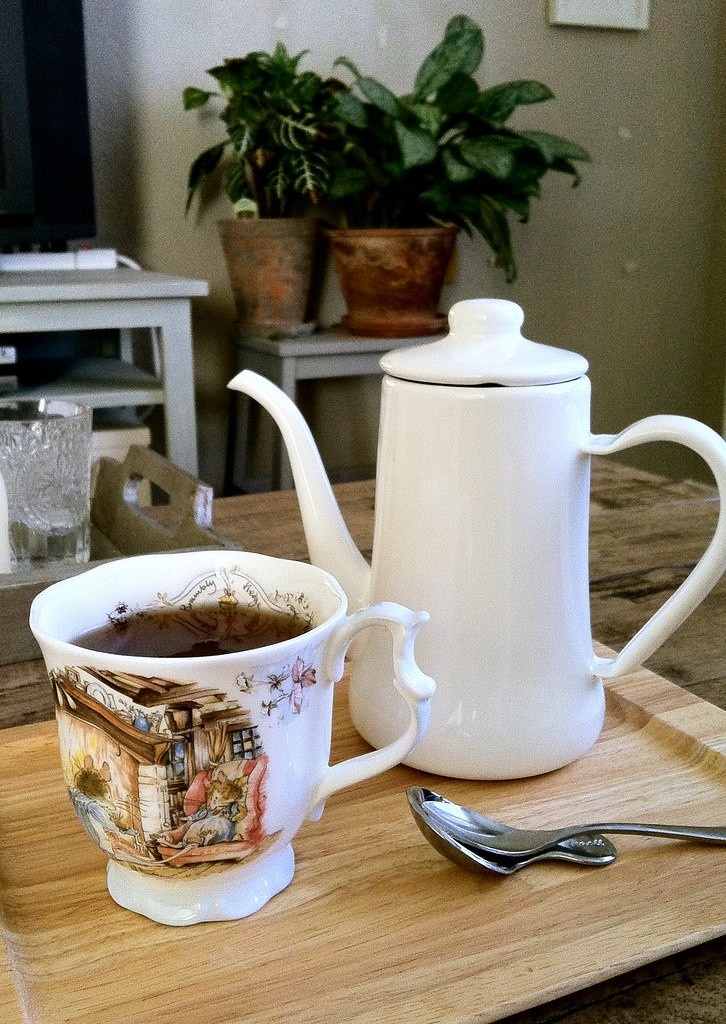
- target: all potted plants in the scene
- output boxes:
[182,42,350,340]
[331,15,593,339]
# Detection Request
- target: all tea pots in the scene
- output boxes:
[223,299,726,781]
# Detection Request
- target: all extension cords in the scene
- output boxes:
[0,250,118,271]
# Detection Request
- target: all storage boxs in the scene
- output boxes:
[90,408,152,507]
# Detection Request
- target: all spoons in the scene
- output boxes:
[422,800,726,858]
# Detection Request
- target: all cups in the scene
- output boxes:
[0,399,96,575]
[29,551,436,926]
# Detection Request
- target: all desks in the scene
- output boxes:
[0,267,210,505]
[0,455,726,1024]
[215,324,448,497]
